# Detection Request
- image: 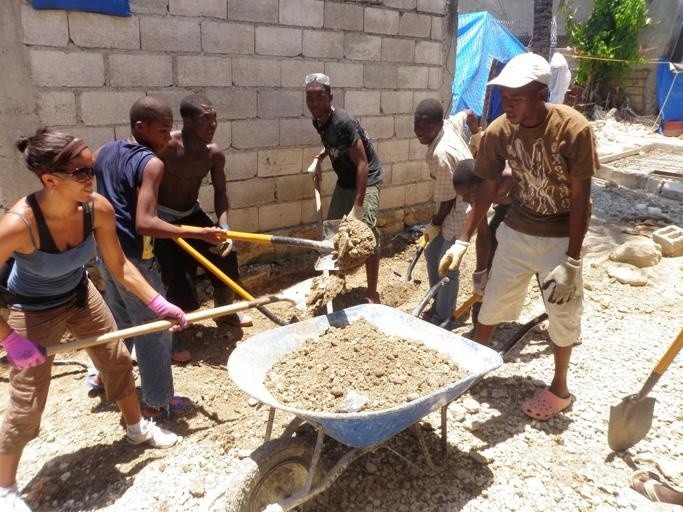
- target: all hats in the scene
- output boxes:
[486,52,552,89]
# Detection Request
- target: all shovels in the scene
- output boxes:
[173,237,301,326]
[0,275,344,363]
[173,219,377,271]
[609,329,683,452]
[381,233,429,308]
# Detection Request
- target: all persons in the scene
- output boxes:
[449,156,519,337]
[88,95,190,419]
[1,126,187,512]
[304,72,383,306]
[154,95,252,363]
[408,97,483,331]
[439,53,599,426]
[628,466,681,506]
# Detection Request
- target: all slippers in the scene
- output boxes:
[141,395,193,420]
[172,349,191,362]
[521,386,572,421]
[213,312,252,327]
[87,369,138,389]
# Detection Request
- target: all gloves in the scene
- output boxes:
[472,268,488,302]
[217,224,233,257]
[307,156,323,182]
[0,330,46,370]
[438,239,470,278]
[415,219,442,250]
[542,253,582,305]
[347,207,363,222]
[146,293,187,333]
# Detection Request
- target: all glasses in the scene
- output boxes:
[305,73,329,86]
[70,165,95,184]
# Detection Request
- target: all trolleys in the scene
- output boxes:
[224,278,551,511]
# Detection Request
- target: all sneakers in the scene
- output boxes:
[125,417,177,449]
[1,483,31,512]
